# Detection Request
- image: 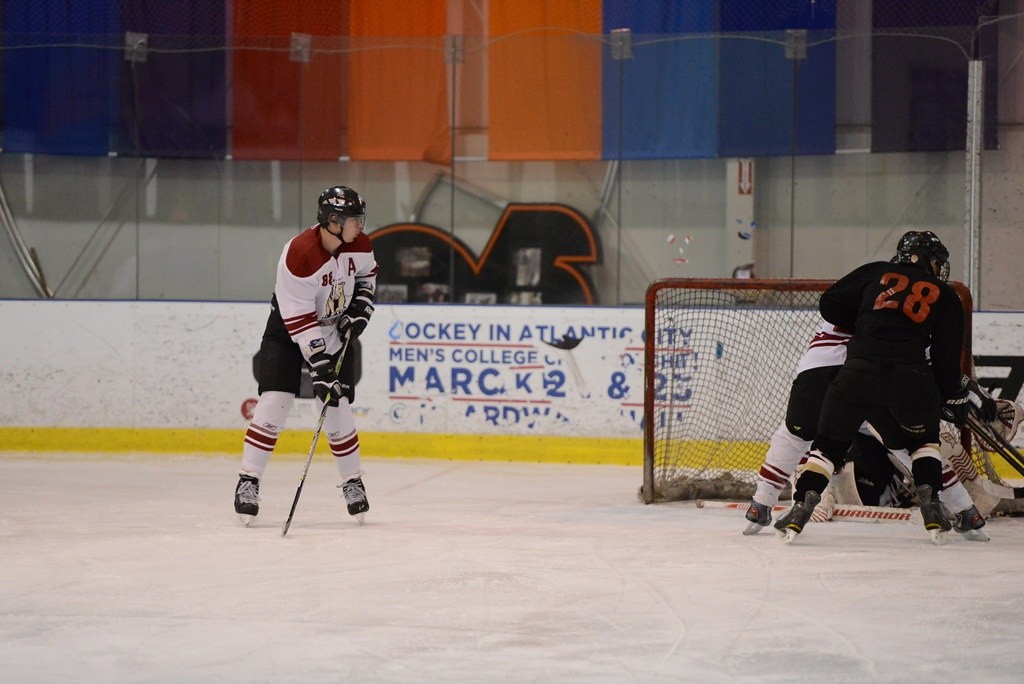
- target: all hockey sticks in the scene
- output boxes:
[943,401,1024,477]
[282,330,353,533]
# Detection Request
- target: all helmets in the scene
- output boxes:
[316,186,366,225]
[896,229,949,275]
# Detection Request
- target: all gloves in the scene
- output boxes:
[337,298,375,342]
[960,375,997,423]
[306,353,342,408]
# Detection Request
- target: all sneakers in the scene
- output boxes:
[336,472,370,526]
[775,490,822,543]
[915,483,952,546]
[233,473,260,527]
[950,505,990,543]
[741,499,773,535]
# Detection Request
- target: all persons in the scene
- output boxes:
[235,184,380,527]
[741,231,997,545]
